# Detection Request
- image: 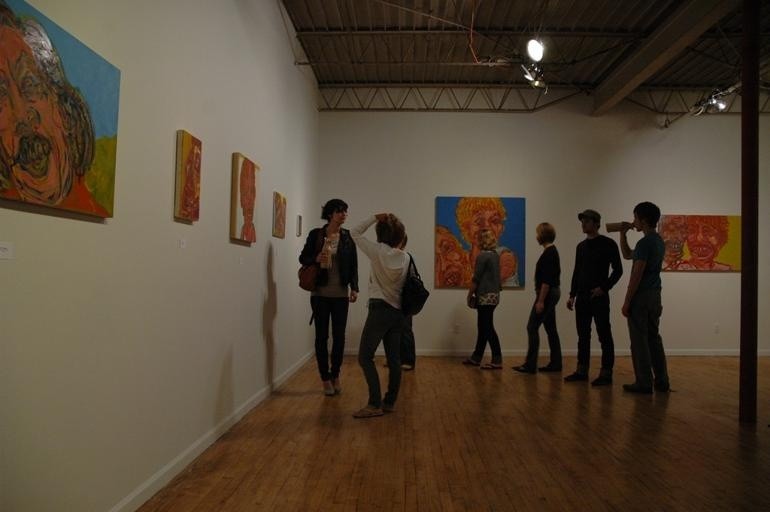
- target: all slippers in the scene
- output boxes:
[353,406,384,418]
[462,358,481,366]
[382,402,395,412]
[480,364,503,370]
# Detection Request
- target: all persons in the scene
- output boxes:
[564,209,623,385]
[349,213,412,418]
[513,223,562,374]
[298,199,359,396]
[384,233,415,371]
[606,202,670,396]
[462,230,502,370]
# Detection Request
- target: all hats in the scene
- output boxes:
[578,210,601,221]
[477,227,498,251]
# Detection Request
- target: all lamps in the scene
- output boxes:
[527,38,544,62]
[522,64,548,91]
[708,98,729,112]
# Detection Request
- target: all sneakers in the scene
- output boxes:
[564,372,588,381]
[622,383,653,395]
[654,377,669,391]
[592,377,613,385]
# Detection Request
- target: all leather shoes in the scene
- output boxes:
[539,364,563,372]
[511,366,537,374]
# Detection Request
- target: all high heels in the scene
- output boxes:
[331,374,342,392]
[322,376,334,395]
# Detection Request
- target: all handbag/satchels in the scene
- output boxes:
[298,264,318,291]
[467,296,478,309]
[400,273,430,315]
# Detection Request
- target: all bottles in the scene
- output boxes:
[606,222,633,233]
[319,237,330,269]
